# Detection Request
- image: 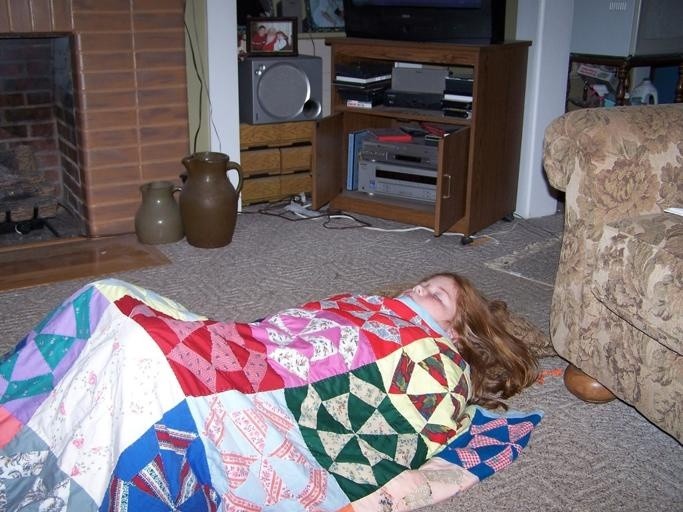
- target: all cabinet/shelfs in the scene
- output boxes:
[623,93,641,106]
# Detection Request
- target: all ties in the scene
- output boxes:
[343,0,518,44]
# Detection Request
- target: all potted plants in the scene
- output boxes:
[565,53,630,113]
[304,0,346,32]
[245,16,299,58]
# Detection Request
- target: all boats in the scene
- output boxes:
[238,54,322,124]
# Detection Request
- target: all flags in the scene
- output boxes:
[179,152,243,248]
[135,180,183,244]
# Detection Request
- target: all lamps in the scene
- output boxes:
[543,102,683,446]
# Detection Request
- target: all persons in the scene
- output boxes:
[238,25,293,54]
[393,272,543,412]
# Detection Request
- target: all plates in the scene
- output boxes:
[312,35,531,246]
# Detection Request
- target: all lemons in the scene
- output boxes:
[133,180,185,245]
[179,152,242,250]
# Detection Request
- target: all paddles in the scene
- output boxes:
[483,230,563,288]
